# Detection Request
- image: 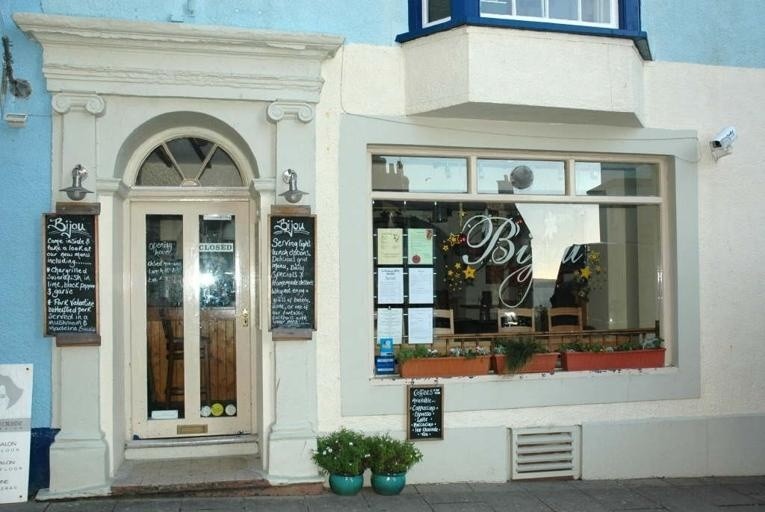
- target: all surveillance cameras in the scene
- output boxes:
[709,127,737,150]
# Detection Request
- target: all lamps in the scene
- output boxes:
[277,168,310,206]
[59,163,97,202]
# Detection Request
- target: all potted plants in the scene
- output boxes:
[366,426,425,497]
[309,423,376,495]
[396,331,672,382]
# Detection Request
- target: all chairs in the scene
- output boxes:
[158,305,214,407]
[496,305,537,335]
[545,305,586,338]
[430,307,454,343]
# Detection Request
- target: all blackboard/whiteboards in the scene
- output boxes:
[147,242,182,306]
[200,240,235,307]
[267,214,317,332]
[42,212,99,336]
[407,385,444,441]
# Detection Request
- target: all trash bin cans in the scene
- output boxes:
[28,428,60,488]
